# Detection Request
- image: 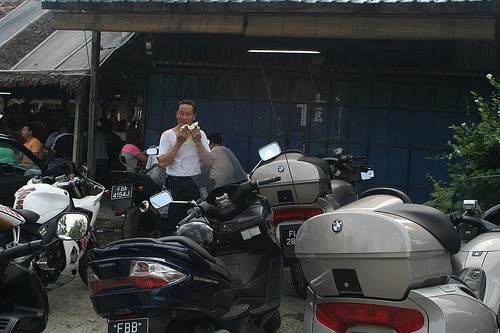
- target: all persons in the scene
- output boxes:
[0,98,123,182]
[121,99,247,221]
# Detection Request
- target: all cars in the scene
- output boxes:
[0,133,56,209]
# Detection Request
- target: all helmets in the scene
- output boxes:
[176,221,224,258]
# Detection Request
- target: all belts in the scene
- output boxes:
[167,176,192,181]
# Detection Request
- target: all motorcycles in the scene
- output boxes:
[103,146,169,239]
[10,147,105,292]
[0,203,91,333]
[83,142,285,333]
[250,136,376,299]
[294,173,500,333]
